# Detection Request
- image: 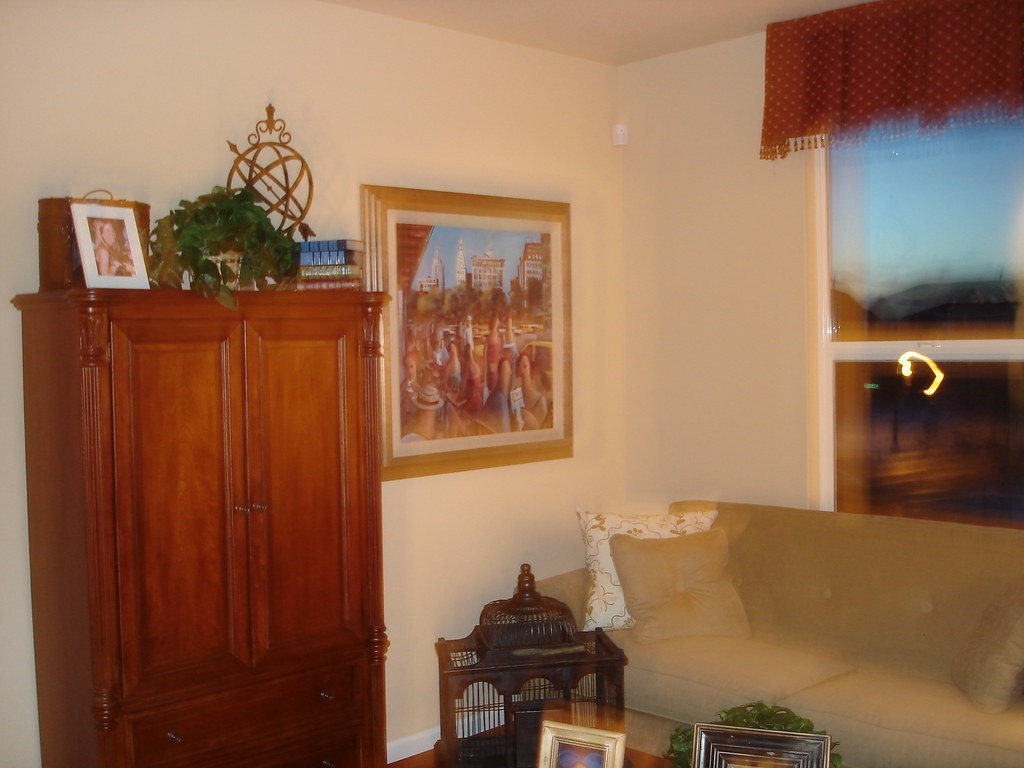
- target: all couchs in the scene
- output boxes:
[514,500,1024,767]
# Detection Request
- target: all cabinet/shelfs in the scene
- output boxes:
[11,291,393,768]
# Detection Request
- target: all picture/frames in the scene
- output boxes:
[535,720,628,768]
[359,183,574,482]
[690,722,832,767]
[62,195,153,292]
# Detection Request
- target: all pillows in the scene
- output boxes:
[576,507,718,631]
[609,529,752,643]
[952,582,1024,711]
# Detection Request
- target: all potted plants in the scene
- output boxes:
[148,186,301,310]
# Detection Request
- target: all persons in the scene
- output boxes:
[89,217,136,277]
